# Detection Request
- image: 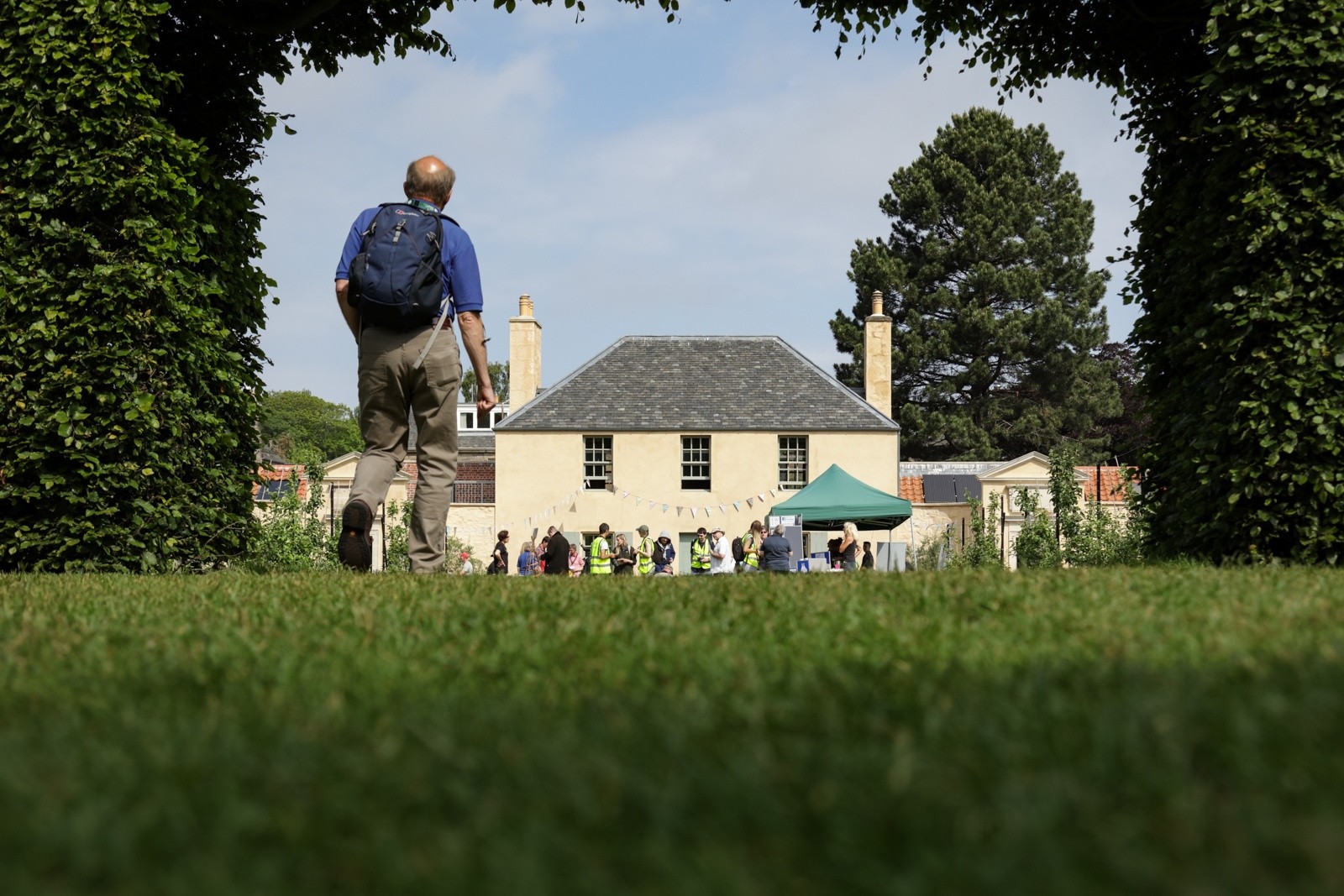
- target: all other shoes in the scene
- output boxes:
[337,499,374,574]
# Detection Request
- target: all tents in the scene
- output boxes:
[765,463,917,570]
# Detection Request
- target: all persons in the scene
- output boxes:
[690,528,715,575]
[651,530,675,576]
[459,553,473,577]
[860,541,873,572]
[611,534,637,575]
[709,526,732,573]
[633,525,654,576]
[569,544,585,577]
[334,156,496,574]
[490,531,509,575]
[839,522,862,572]
[538,526,570,576]
[517,536,551,576]
[739,521,793,575]
[590,523,616,574]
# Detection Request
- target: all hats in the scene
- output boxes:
[635,525,648,533]
[709,526,725,536]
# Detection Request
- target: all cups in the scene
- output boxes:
[834,560,840,568]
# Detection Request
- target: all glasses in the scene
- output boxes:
[761,531,768,534]
[697,535,704,537]
[505,535,510,539]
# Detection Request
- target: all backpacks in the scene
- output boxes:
[487,561,495,575]
[347,202,461,336]
[731,534,752,562]
